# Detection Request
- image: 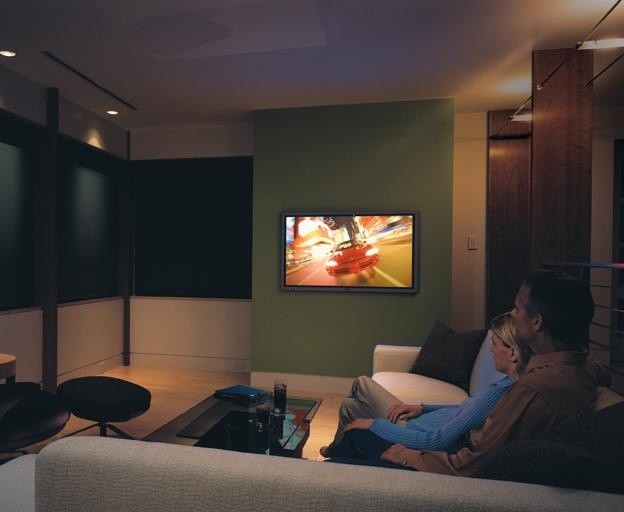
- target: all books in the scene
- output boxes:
[212,382,269,403]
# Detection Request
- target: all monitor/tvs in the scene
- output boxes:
[279,208,420,296]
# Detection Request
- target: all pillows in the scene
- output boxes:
[408,320,488,394]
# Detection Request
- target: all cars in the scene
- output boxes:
[326,240,380,275]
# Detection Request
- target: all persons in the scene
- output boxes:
[325,265,599,478]
[319,311,533,458]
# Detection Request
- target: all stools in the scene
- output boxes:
[0,354,17,385]
[0,376,151,465]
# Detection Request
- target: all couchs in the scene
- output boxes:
[0,342,624,511]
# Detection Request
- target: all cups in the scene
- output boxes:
[273,375,288,415]
[255,392,271,423]
